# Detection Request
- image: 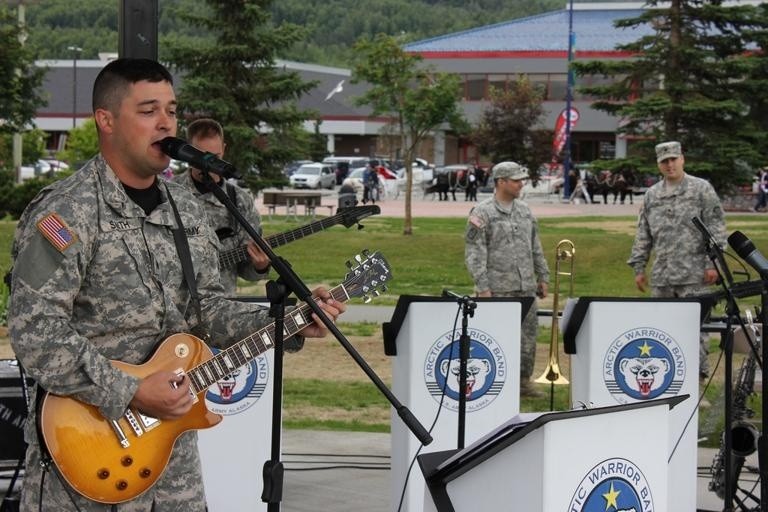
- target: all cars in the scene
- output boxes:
[288,162,337,190]
[346,167,403,199]
[394,158,435,186]
[434,164,490,186]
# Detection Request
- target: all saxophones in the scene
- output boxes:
[708,310,760,499]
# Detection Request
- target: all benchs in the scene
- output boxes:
[263,191,336,218]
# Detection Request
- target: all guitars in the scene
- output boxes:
[40,248,393,505]
[213,199,381,268]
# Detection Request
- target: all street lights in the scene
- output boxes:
[66,45,84,129]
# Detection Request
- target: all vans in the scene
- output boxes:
[324,156,378,184]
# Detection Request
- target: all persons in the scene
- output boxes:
[464,162,552,396]
[360,159,392,203]
[7,60,345,512]
[168,118,274,301]
[753,166,768,210]
[626,142,730,408]
[463,164,478,202]
[335,162,346,184]
[569,161,580,196]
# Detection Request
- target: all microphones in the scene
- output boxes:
[160,133,234,183]
[440,286,476,318]
[730,222,768,276]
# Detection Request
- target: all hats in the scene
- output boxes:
[492,160,530,182]
[655,140,682,163]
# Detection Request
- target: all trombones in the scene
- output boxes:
[535,238,577,393]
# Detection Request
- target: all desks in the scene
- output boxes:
[285,193,320,222]
